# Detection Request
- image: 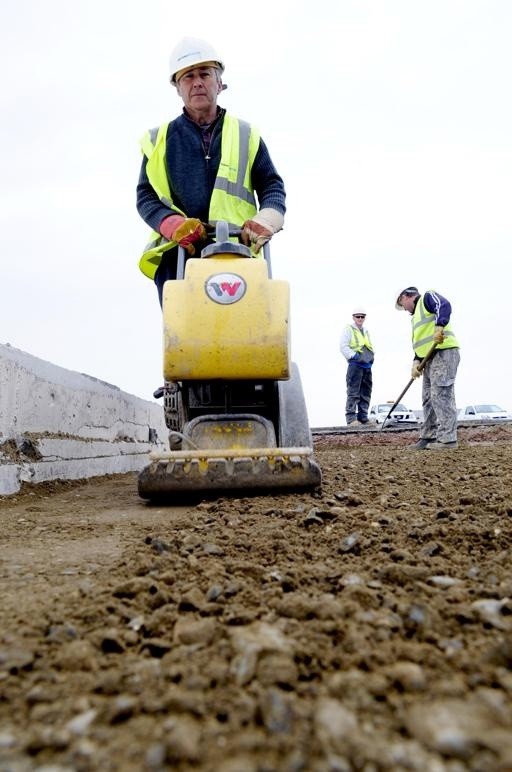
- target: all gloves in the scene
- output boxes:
[159,216,206,255]
[433,327,444,343]
[240,209,284,254]
[411,360,421,379]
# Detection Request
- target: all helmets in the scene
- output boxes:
[394,286,418,311]
[168,38,225,84]
[352,309,366,315]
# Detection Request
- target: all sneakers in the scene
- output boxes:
[426,442,459,450]
[408,439,437,450]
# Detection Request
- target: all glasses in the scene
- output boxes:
[355,316,364,319]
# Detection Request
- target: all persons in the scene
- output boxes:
[339,309,374,425]
[134,47,286,312]
[393,279,459,450]
